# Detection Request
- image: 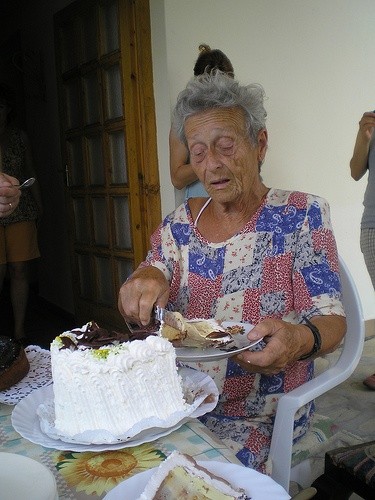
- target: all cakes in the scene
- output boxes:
[158,307,235,350]
[0,335,31,393]
[52,320,189,437]
[136,449,251,500]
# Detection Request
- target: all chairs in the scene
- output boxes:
[267,259,365,496]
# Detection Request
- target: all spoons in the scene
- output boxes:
[13,177,35,190]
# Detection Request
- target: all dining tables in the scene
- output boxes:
[0,353,292,500]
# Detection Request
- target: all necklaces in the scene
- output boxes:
[194,197,213,228]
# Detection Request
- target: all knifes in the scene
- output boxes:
[152,304,185,332]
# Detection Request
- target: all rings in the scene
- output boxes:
[9,203,14,209]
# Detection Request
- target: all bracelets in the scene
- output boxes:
[297,317,321,362]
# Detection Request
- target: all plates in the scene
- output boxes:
[11,367,220,453]
[102,461,291,500]
[175,322,264,363]
[0,452,58,500]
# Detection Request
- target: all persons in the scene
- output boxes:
[350,110,375,390]
[0,92,44,347]
[116,68,348,474]
[169,44,236,206]
